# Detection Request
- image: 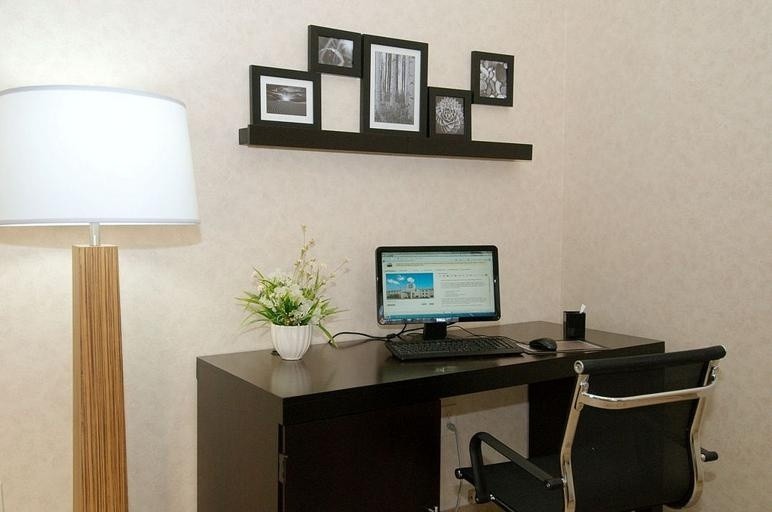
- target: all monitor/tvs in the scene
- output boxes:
[374,244,501,340]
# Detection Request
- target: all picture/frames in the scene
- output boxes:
[361,34,428,136]
[308,25,361,77]
[250,65,321,129]
[428,87,471,140]
[471,51,514,106]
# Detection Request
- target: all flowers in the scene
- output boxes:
[234,223,351,348]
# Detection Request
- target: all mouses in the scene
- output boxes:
[529,336,558,352]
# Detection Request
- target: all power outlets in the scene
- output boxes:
[441,405,457,434]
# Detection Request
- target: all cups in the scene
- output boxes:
[563,312,586,342]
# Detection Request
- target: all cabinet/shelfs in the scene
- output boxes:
[271,323,312,360]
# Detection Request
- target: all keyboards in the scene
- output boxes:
[383,335,523,362]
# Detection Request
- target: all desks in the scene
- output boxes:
[197,320,665,511]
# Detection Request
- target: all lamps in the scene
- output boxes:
[0,86,200,512]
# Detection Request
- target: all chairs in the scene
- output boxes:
[455,344,726,512]
